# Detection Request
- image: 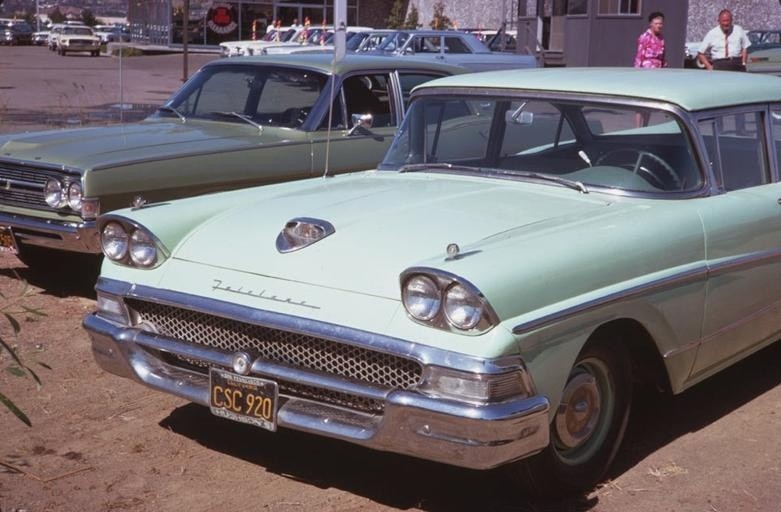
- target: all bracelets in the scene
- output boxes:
[742,62,747,66]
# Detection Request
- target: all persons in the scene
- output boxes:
[696,9,754,137]
[265,15,311,33]
[632,12,667,127]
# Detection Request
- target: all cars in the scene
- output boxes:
[685,29,781,72]
[81,68,781,495]
[0,54,605,278]
[0,18,128,57]
